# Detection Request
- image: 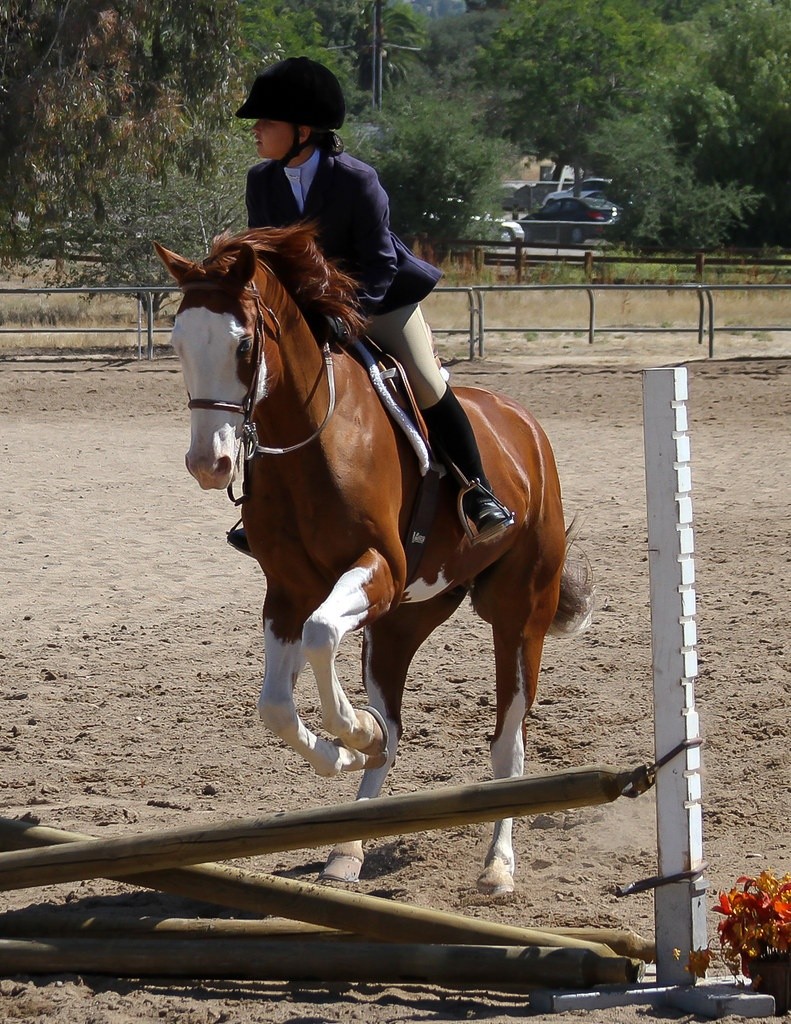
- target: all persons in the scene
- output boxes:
[228,57,513,558]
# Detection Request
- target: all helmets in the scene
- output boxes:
[234,56,346,131]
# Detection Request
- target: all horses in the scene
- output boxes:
[152,215,595,893]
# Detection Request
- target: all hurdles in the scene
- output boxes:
[1,369,779,1016]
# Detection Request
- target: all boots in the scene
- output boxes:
[228,526,252,554]
[422,381,512,534]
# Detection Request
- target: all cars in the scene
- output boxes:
[426,196,525,244]
[543,179,625,207]
[515,199,623,244]
